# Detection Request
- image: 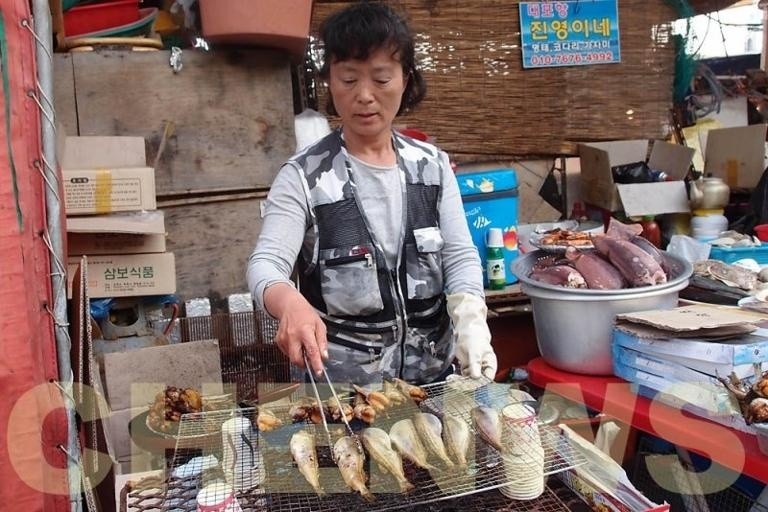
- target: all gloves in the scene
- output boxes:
[446,292,498,391]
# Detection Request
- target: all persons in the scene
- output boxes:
[242,0,501,472]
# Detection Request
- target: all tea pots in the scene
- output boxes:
[689,172,731,209]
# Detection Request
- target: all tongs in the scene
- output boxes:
[303,346,367,467]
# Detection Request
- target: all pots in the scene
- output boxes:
[509,245,696,378]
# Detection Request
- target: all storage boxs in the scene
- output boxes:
[703,123,767,193]
[104,338,224,474]
[455,168,520,290]
[198,0,315,65]
[577,138,696,218]
[59,135,177,300]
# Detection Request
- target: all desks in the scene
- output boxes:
[526,356,768,512]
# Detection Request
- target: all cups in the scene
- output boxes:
[220,414,267,490]
[195,483,244,512]
[486,228,505,247]
[497,403,545,501]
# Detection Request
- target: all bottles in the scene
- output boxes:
[641,215,662,250]
[486,248,506,290]
